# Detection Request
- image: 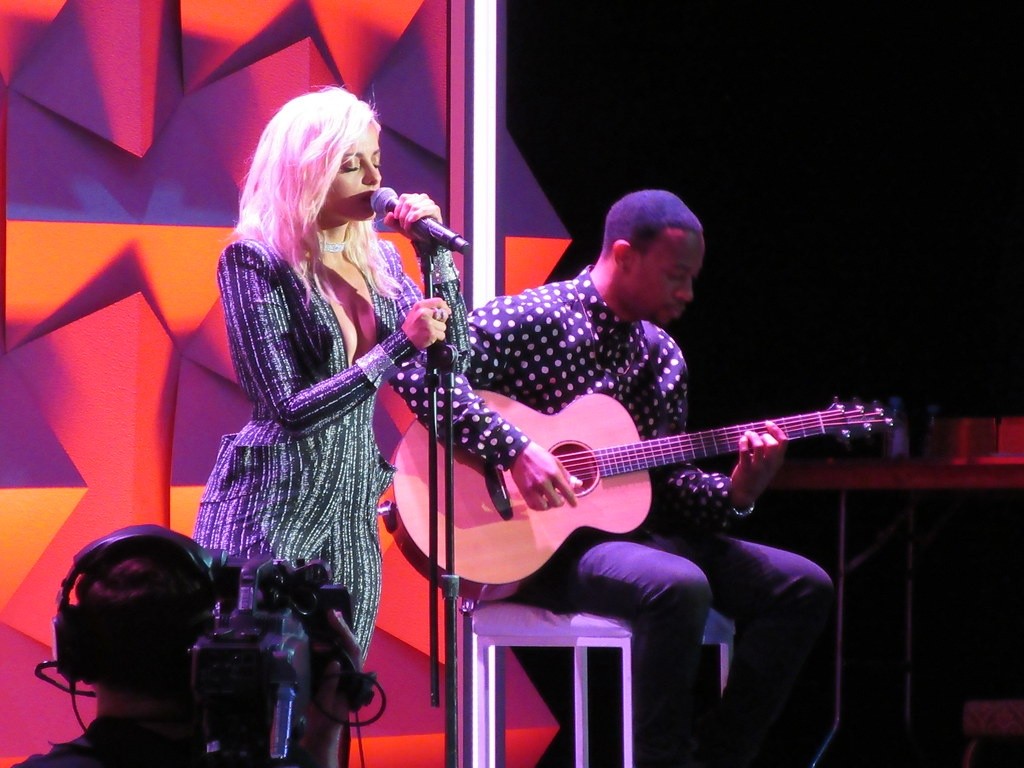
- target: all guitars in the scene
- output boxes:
[375,389,898,604]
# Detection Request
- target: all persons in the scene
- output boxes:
[387,188,837,768]
[191,89,474,768]
[6,524,366,768]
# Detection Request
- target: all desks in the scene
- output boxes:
[781,458,1024,768]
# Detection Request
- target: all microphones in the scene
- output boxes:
[370,187,470,255]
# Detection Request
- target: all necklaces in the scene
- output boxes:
[572,279,637,375]
[318,238,347,253]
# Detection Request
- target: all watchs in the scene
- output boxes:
[732,501,755,517]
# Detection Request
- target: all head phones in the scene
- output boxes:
[55,525,220,686]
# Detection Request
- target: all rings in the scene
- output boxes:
[753,454,766,463]
[430,307,447,322]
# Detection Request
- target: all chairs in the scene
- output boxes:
[465,600,737,768]
